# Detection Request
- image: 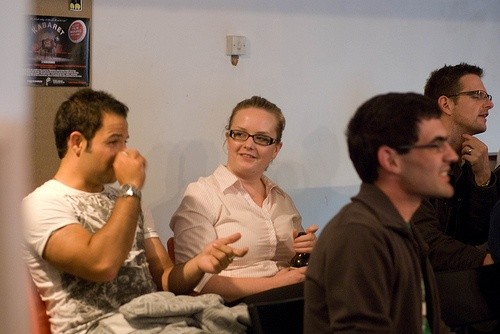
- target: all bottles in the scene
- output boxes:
[288,231,312,269]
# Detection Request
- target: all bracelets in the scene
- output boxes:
[482,180,491,187]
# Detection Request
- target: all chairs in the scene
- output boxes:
[25,266,52,334]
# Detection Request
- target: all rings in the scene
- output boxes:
[468,147,473,154]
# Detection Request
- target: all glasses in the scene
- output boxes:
[230,129,277,146]
[400,139,446,152]
[449,90,492,102]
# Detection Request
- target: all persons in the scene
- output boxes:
[303,63,500,334]
[169,95,320,334]
[21,90,249,334]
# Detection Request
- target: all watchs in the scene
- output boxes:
[119,183,142,199]
